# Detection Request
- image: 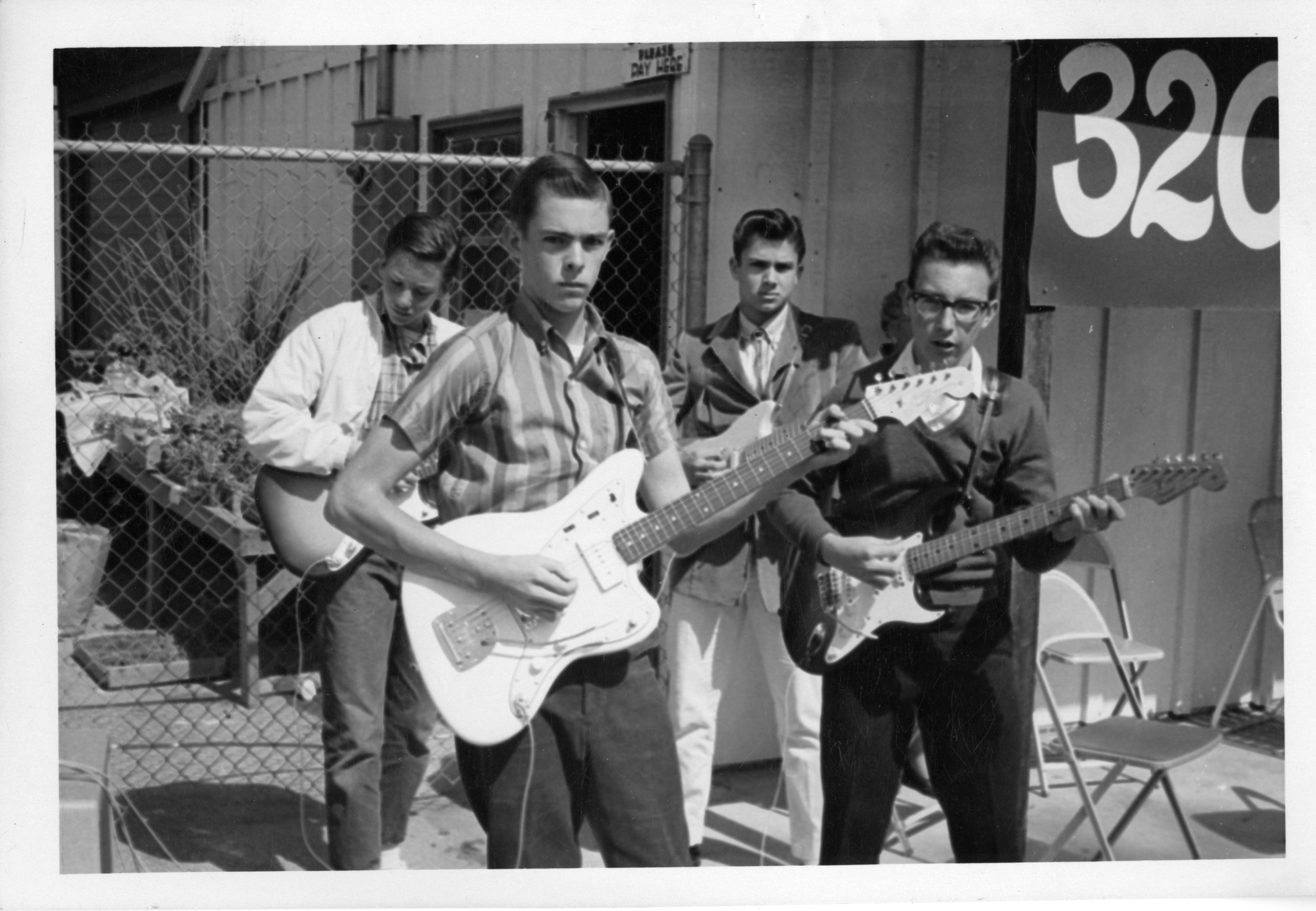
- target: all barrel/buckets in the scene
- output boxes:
[57,517,112,637]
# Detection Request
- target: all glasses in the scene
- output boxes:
[911,291,991,323]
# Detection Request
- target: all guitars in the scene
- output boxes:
[776,450,1233,677]
[254,441,441,581]
[676,397,812,495]
[398,356,975,747]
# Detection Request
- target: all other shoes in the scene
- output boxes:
[384,861,406,869]
[690,849,701,866]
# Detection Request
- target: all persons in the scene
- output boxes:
[322,149,879,869]
[663,206,871,866]
[241,212,466,871]
[763,219,1126,865]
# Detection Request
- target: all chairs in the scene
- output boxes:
[1209,493,1284,731]
[1020,530,1223,861]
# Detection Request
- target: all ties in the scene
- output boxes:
[754,331,768,398]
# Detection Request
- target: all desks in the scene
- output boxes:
[103,442,320,710]
